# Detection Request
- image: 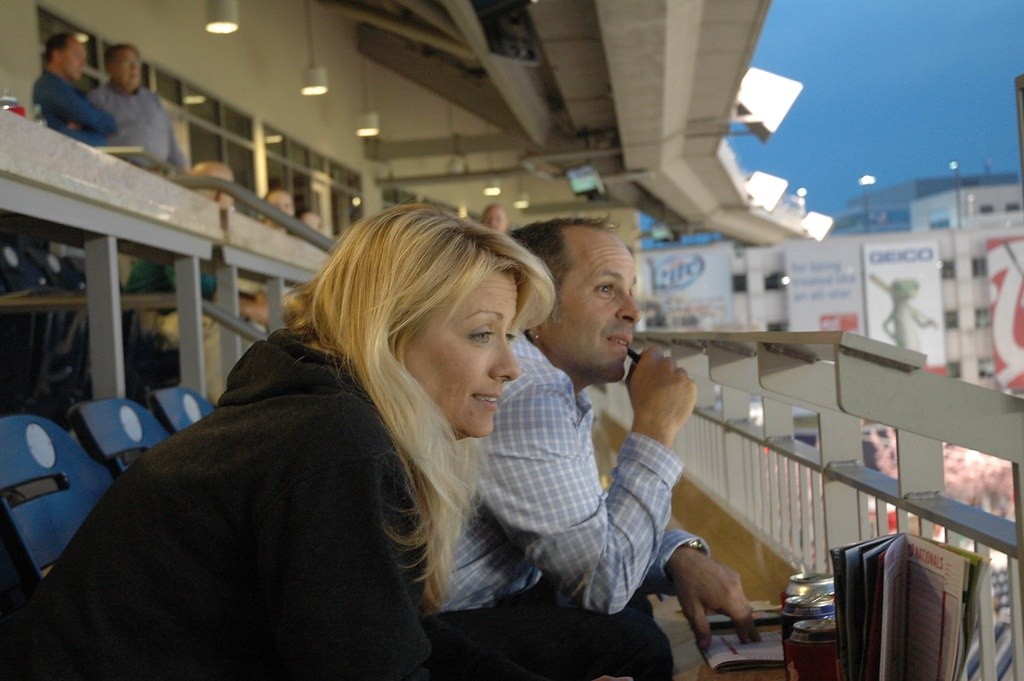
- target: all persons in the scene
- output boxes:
[479,201,511,232]
[0,200,632,681]
[81,45,187,172]
[127,159,325,320]
[30,32,120,148]
[420,215,758,649]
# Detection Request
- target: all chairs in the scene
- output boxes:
[68,398,168,478]
[149,387,212,435]
[0,415,115,587]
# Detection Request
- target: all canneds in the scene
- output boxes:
[780,572,845,681]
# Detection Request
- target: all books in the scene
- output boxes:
[827,532,993,681]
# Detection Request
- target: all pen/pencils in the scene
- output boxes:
[720,635,739,655]
[626,346,641,364]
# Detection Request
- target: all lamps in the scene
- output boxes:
[447,97,469,175]
[299,0,328,96]
[748,171,790,212]
[802,212,834,239]
[733,66,803,133]
[480,123,502,196]
[205,0,239,34]
[355,33,379,136]
[513,163,531,209]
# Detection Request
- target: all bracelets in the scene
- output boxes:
[686,536,708,552]
